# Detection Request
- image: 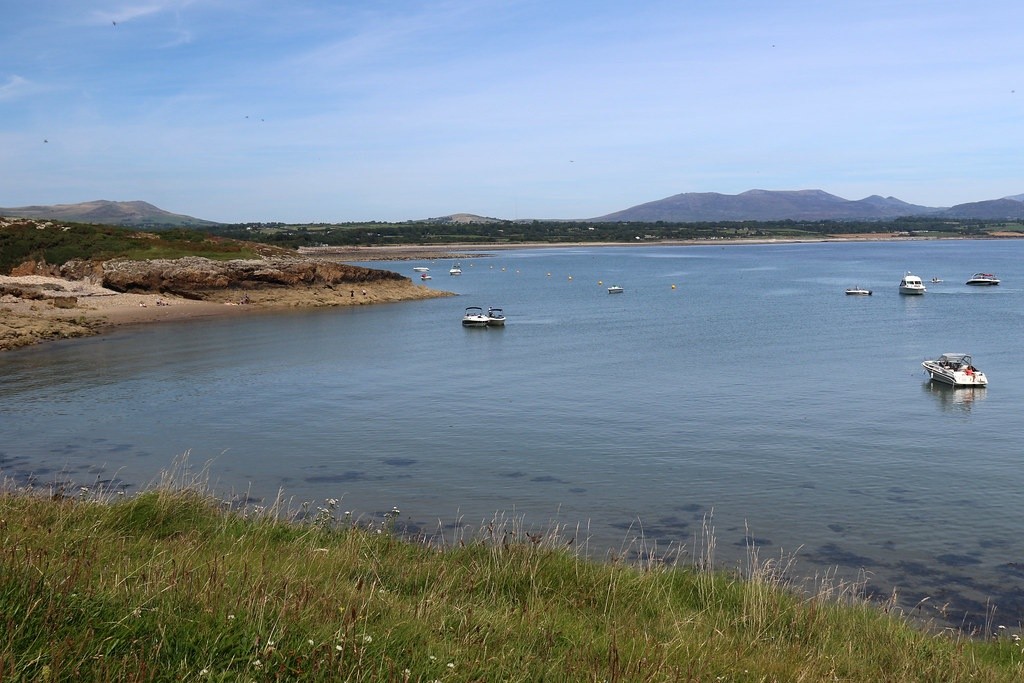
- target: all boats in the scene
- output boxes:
[462,306,487,327]
[608,285,624,294]
[487,308,506,325]
[967,270,1000,288]
[931,277,944,283]
[414,266,429,273]
[898,275,926,295]
[421,273,434,282]
[920,350,989,392]
[847,286,874,296]
[450,266,462,275]
